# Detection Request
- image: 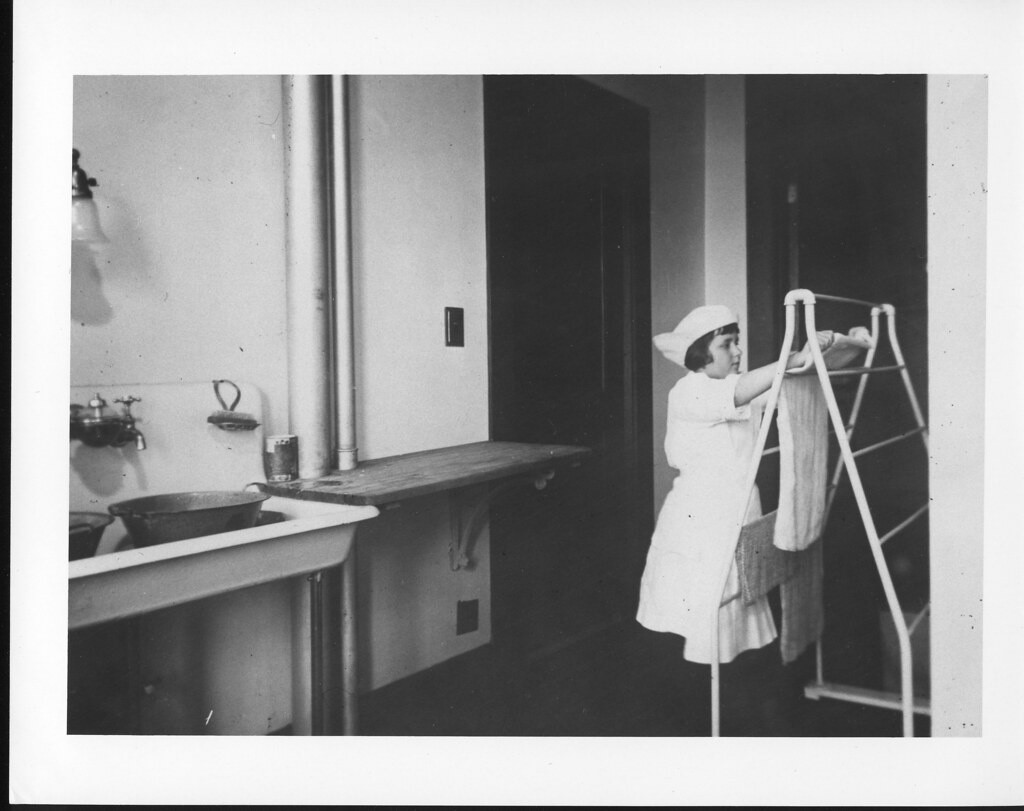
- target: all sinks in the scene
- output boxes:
[69,494,381,631]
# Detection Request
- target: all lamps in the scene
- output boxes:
[72,148,113,244]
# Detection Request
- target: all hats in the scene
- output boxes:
[650,303,738,368]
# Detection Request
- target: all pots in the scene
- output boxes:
[69,511,116,561]
[107,483,273,548]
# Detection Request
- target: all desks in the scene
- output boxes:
[269,440,592,573]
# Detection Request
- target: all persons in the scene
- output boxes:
[635,305,870,737]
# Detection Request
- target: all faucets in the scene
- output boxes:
[113,394,147,452]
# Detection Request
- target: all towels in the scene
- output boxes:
[734,328,876,667]
